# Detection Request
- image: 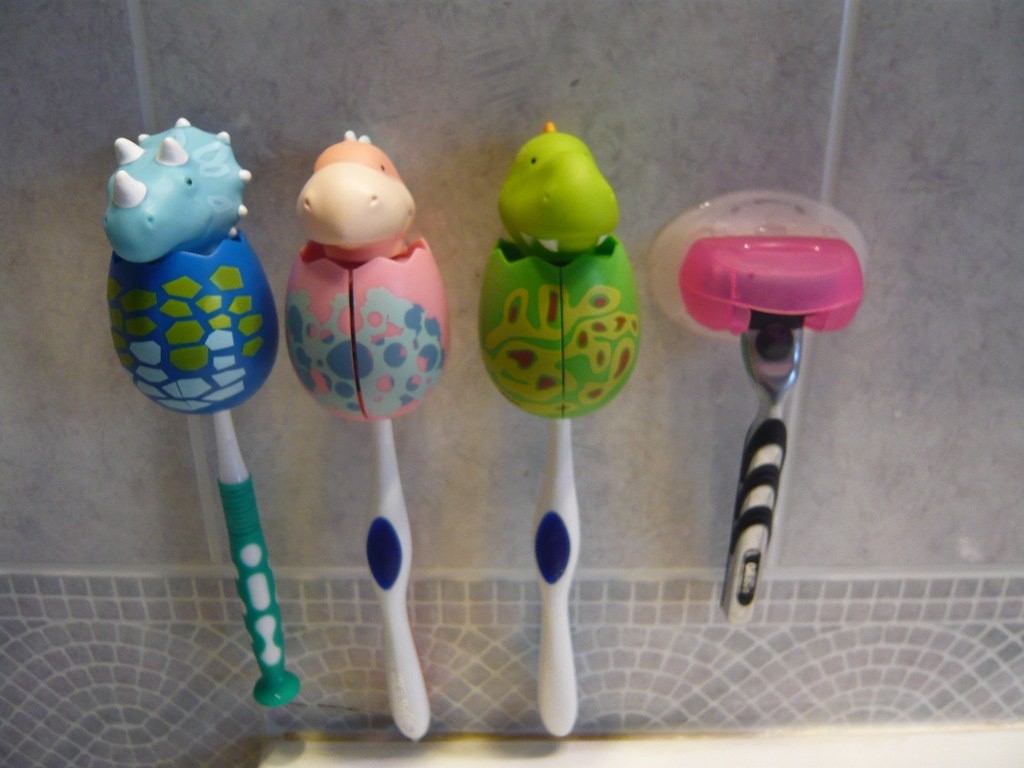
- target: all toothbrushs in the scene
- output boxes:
[100,116,302,706]
[478,122,640,734]
[282,132,450,742]
